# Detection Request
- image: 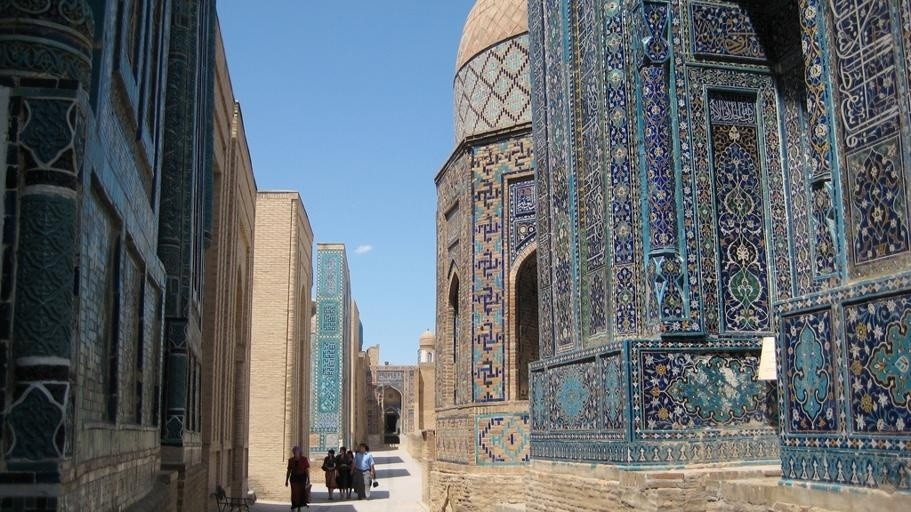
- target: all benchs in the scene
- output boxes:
[209,485,254,512]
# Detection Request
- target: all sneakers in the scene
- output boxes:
[327,493,370,500]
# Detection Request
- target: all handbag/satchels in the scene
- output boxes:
[306,485,312,503]
[373,482,378,487]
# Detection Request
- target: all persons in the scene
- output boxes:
[285,445,310,512]
[323,442,377,500]
